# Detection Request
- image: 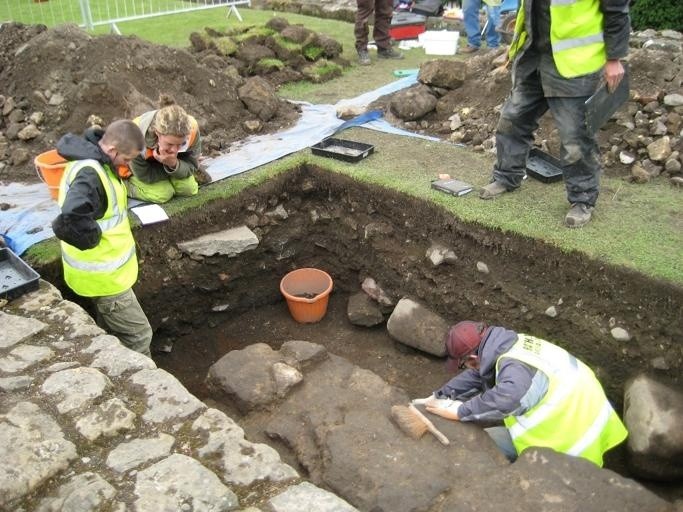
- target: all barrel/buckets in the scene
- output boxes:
[33,149,69,201]
[278,267,334,326]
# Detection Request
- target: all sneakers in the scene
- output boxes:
[193,168,211,185]
[359,51,370,66]
[565,202,594,228]
[479,181,507,200]
[484,45,499,52]
[460,45,478,53]
[377,48,403,59]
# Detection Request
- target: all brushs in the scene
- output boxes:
[390,404,450,446]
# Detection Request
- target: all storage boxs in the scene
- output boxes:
[387,12,426,41]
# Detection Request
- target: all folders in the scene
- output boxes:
[583,67,630,139]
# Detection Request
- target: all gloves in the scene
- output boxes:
[424,397,463,420]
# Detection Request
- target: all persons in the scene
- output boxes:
[122,95,212,203]
[51,119,153,359]
[353,0,404,65]
[478,0,631,227]
[411,321,628,468]
[458,0,503,53]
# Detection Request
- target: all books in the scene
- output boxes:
[131,204,169,225]
[431,178,474,197]
[584,62,630,136]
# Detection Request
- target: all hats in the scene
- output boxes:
[445,321,486,373]
